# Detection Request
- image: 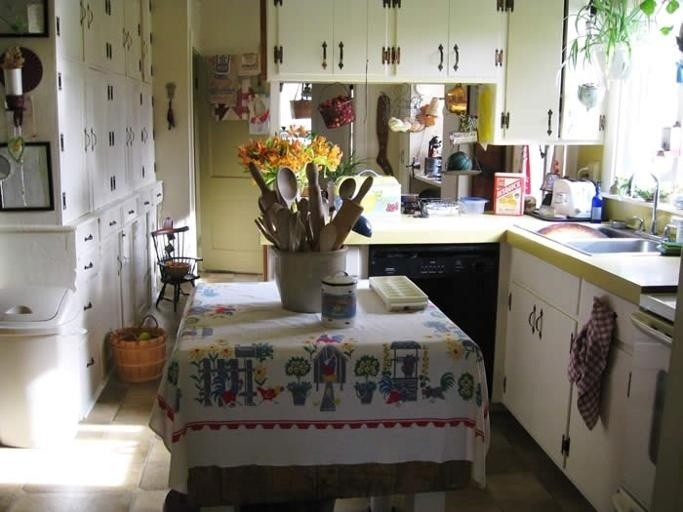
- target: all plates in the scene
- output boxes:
[538,222,610,239]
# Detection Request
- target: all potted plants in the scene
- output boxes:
[556,0,670,79]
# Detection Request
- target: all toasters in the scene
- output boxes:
[550,176,598,218]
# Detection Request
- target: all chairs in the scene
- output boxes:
[149,223,206,312]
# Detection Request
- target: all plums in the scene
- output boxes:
[320,95,354,128]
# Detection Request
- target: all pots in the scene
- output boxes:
[445,84,467,117]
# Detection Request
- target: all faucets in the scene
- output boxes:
[625,168,660,234]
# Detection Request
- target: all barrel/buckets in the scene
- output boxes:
[105,314,168,384]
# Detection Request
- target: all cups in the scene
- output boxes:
[318,269,357,330]
[271,244,348,314]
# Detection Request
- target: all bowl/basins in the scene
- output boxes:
[610,219,628,229]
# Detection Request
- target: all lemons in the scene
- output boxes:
[137,332,150,341]
[165,261,173,265]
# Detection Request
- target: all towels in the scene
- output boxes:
[567,292,618,432]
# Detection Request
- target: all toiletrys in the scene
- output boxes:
[590,180,604,223]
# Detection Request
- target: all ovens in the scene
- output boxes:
[368,243,495,403]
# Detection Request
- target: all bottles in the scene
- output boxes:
[590,178,604,222]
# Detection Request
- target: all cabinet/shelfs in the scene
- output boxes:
[272,1,391,77]
[475,2,607,148]
[563,272,639,512]
[500,239,582,475]
[400,86,443,193]
[396,1,506,78]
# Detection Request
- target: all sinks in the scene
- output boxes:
[561,238,666,258]
[513,221,639,239]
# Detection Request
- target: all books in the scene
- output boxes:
[494,172,525,217]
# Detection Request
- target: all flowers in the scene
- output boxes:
[239,131,343,206]
